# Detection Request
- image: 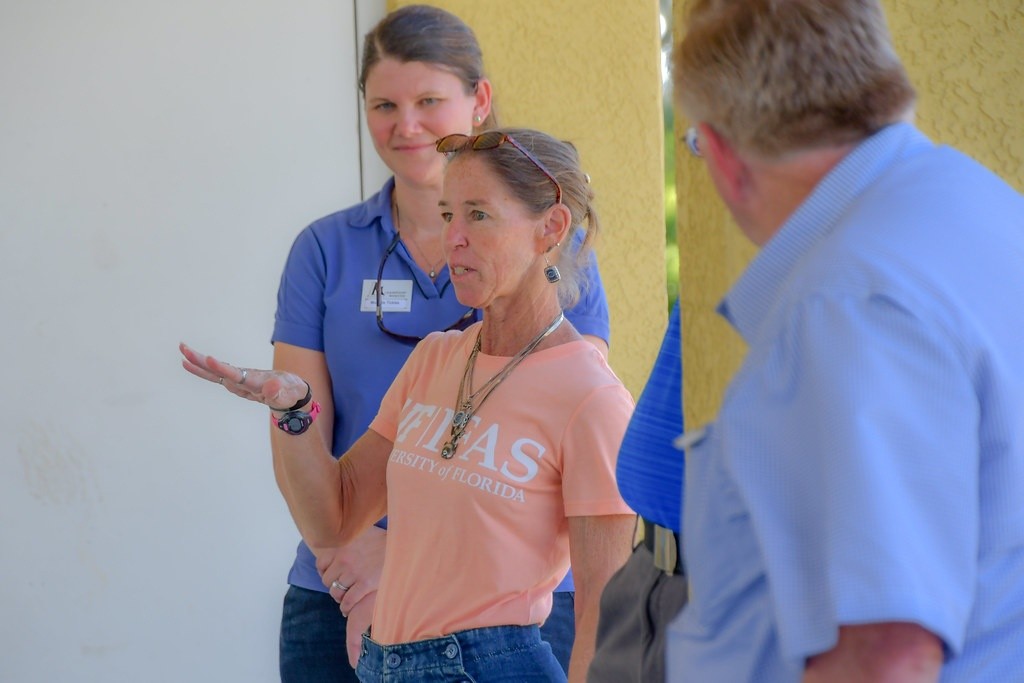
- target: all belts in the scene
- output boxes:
[642,521,685,575]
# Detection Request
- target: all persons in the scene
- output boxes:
[269,5,610,683]
[664,0,1024,683]
[613,295,683,535]
[179,124,640,683]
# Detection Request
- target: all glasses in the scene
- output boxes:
[373,231,478,345]
[688,126,704,157]
[436,131,562,204]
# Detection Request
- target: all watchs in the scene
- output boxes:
[271,401,321,436]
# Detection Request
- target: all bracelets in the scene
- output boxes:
[270,378,312,412]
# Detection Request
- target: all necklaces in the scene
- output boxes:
[406,227,442,278]
[441,308,565,460]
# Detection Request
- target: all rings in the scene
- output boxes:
[219,377,222,383]
[240,370,246,384]
[333,580,348,590]
